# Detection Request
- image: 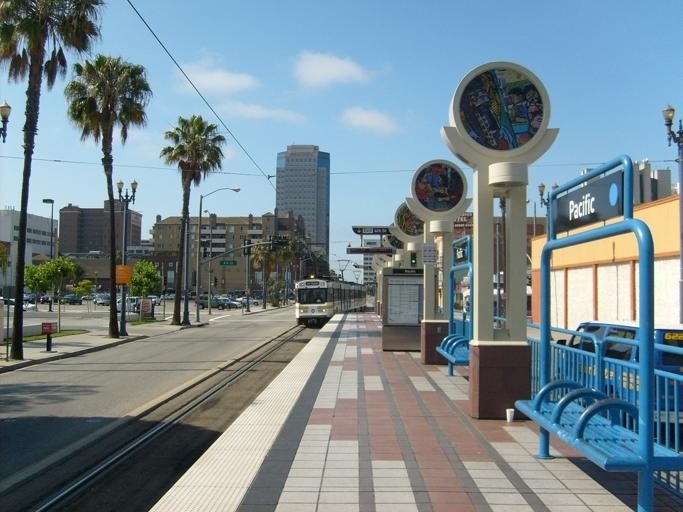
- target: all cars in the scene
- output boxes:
[195,294,259,310]
[0,293,161,312]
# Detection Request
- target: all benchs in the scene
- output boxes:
[436,318,473,376]
[514,326,683,512]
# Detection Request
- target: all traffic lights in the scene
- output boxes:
[411,253,416,266]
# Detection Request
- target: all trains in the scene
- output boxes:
[295,274,366,327]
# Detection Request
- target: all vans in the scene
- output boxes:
[557,321,683,425]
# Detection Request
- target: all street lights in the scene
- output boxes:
[203,210,212,315]
[663,105,683,327]
[195,187,240,323]
[117,180,138,338]
[43,199,54,259]
